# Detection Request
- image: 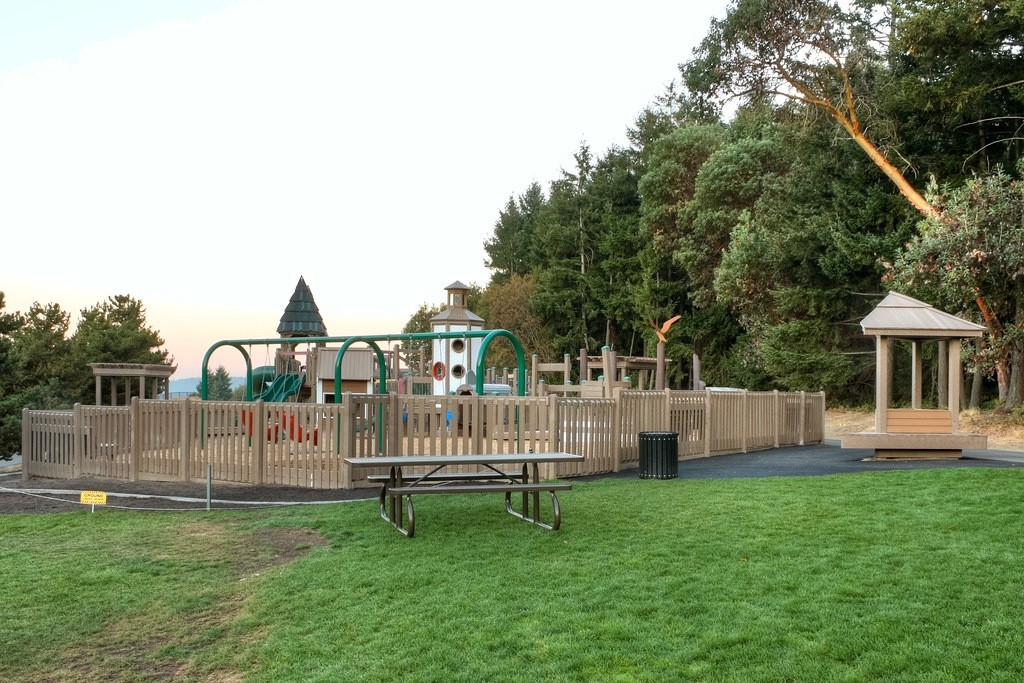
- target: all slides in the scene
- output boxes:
[244,373,311,444]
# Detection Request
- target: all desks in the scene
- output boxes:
[342,452,586,530]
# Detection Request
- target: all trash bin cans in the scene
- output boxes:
[637,431,679,480]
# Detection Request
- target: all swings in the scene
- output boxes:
[385,337,466,439]
[243,345,311,442]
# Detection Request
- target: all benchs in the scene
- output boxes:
[366,470,530,521]
[388,482,572,538]
